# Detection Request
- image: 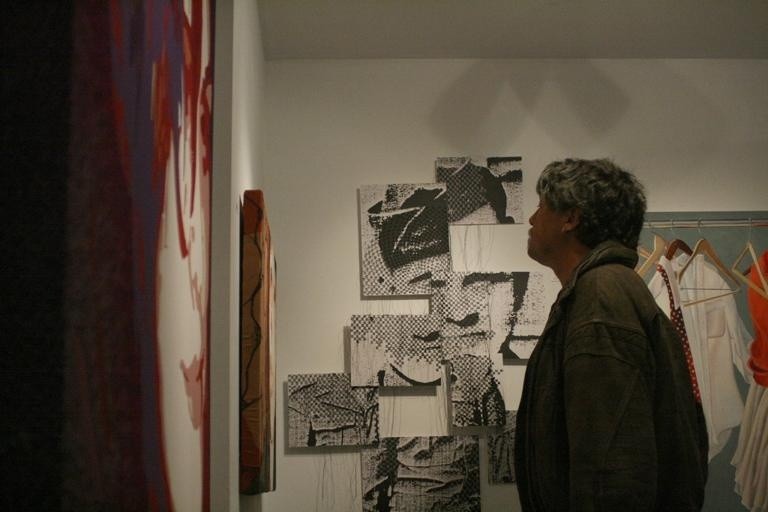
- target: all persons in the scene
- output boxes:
[514,158,708,511]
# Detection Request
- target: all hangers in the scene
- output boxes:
[633,218,768,305]
